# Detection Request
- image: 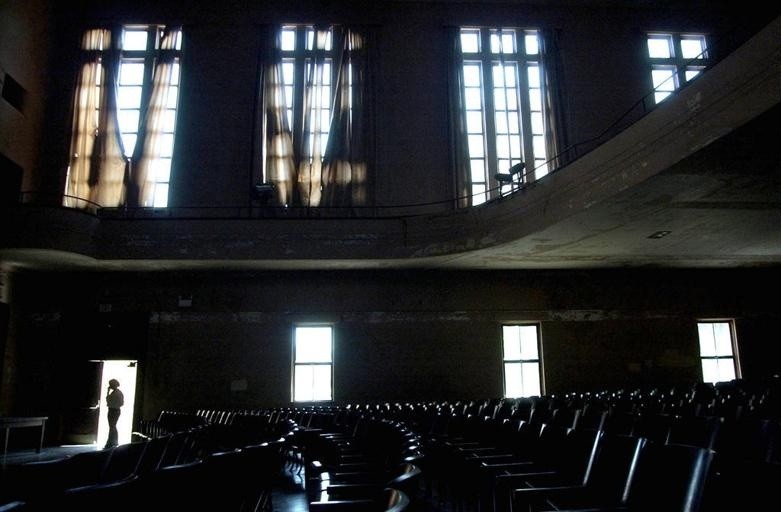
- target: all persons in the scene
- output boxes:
[103,378,125,450]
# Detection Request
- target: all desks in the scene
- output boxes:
[1,416,48,453]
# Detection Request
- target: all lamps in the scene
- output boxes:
[251,182,276,213]
[494,162,529,201]
